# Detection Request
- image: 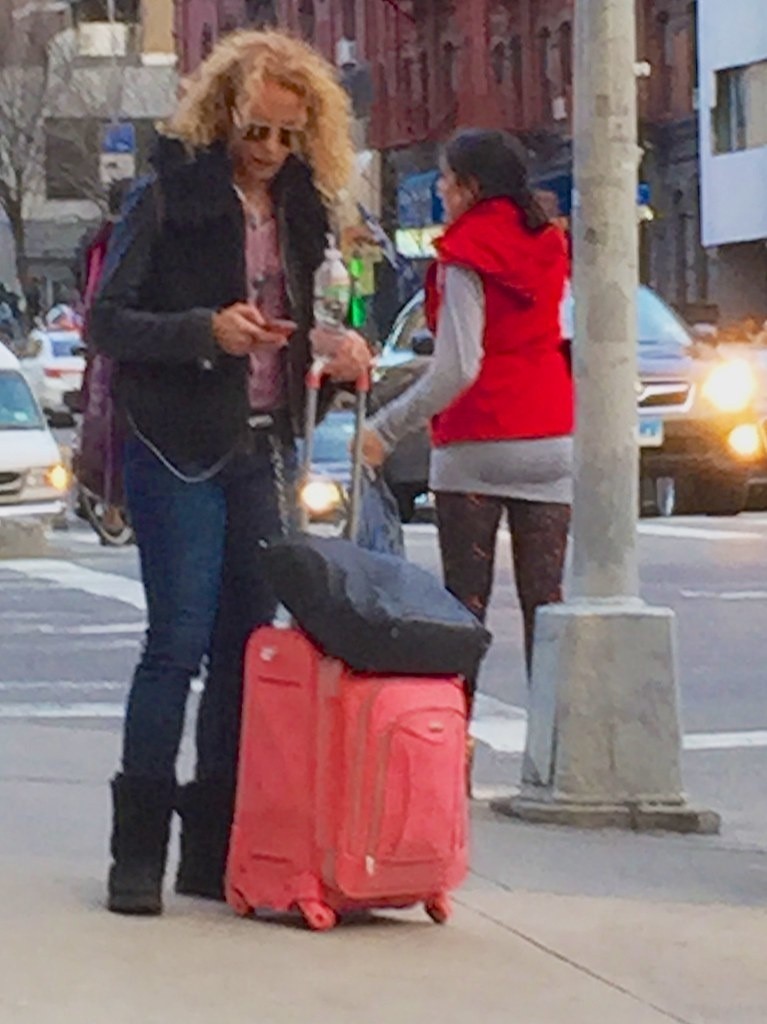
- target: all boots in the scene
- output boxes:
[176,782,236,900]
[105,775,173,915]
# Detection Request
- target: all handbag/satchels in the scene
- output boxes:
[263,534,492,674]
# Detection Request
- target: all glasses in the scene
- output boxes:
[226,98,309,150]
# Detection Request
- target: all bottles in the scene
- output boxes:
[311,249,352,361]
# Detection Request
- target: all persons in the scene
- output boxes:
[347,128,575,799]
[82,30,385,915]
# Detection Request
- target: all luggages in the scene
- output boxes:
[224,356,470,929]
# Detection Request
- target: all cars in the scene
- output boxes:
[295,411,356,524]
[17,321,89,415]
[0,346,71,508]
[365,259,767,517]
